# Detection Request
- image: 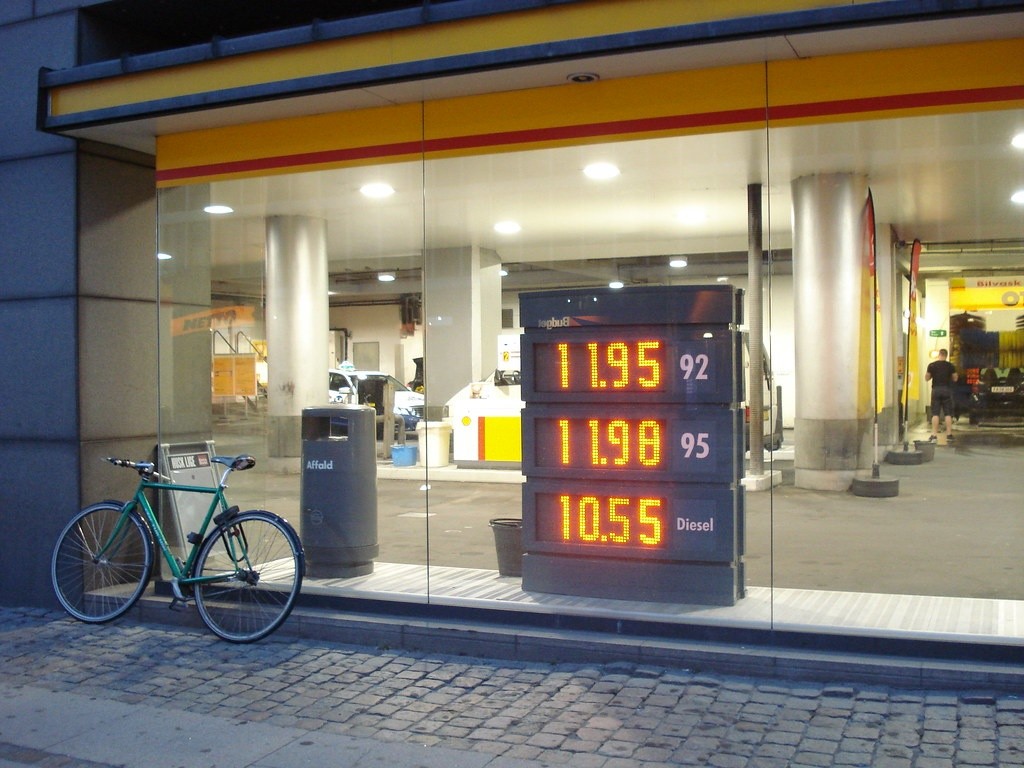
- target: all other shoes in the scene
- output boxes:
[946,435,957,441]
[929,435,937,443]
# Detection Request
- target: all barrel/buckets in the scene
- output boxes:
[417,421,451,468]
[488,519,523,578]
[391,445,416,466]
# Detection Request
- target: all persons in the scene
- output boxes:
[925,349,958,444]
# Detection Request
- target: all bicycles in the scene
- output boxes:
[49,454,305,647]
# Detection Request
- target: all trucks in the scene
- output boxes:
[443,327,784,464]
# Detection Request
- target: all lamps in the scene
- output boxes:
[378,273,396,281]
[669,255,687,268]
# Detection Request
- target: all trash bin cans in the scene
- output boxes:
[299,402,380,578]
[416,421,451,468]
[489,518,524,577]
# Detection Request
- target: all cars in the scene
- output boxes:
[954,366,1024,425]
[332,369,425,423]
